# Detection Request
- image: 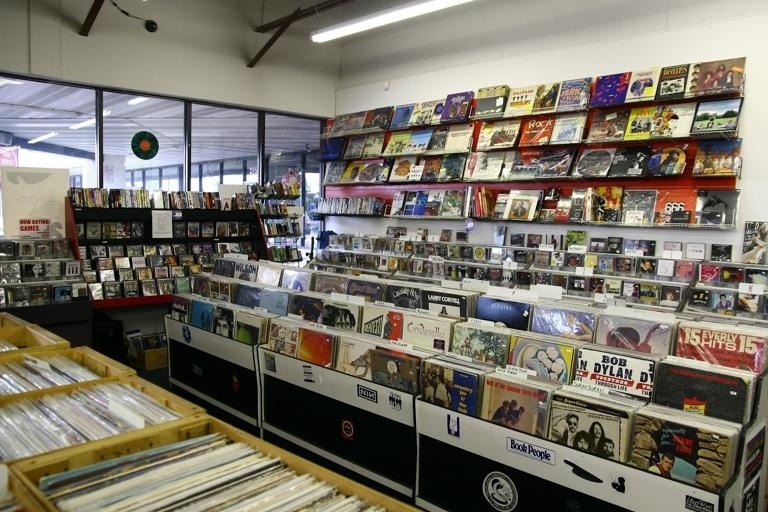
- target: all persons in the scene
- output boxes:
[269,322,298,356]
[492,397,525,428]
[710,149,741,171]
[663,288,678,301]
[641,261,654,273]
[703,63,736,88]
[213,305,235,340]
[592,278,603,293]
[690,63,701,93]
[716,294,731,311]
[648,451,676,478]
[560,312,592,342]
[140,267,150,279]
[387,140,427,153]
[662,80,683,94]
[423,367,452,409]
[562,413,615,459]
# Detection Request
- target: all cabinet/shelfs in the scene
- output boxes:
[316,76,747,232]
[0,311,419,512]
[1,181,304,314]
[165,234,767,511]
